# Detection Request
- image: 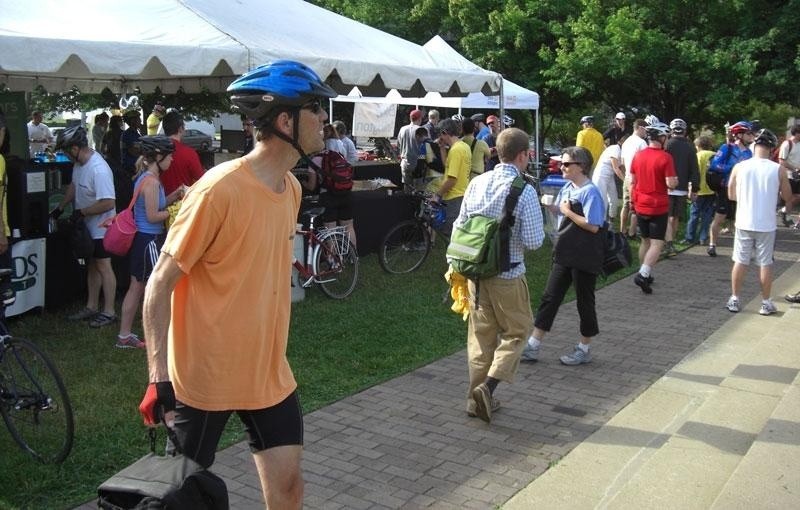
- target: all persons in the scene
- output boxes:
[159,111,204,194]
[619,119,647,240]
[784,291,800,304]
[0,119,12,253]
[630,122,679,294]
[139,61,337,510]
[707,121,753,255]
[422,110,440,139]
[592,135,629,229]
[664,119,700,257]
[414,128,446,248]
[429,119,472,233]
[520,146,605,366]
[396,110,422,190]
[724,120,761,155]
[119,111,141,174]
[576,116,605,169]
[92,112,109,155]
[778,124,800,226]
[333,120,357,160]
[300,149,359,271]
[470,113,488,140]
[48,126,116,327]
[322,124,346,158]
[460,119,490,184]
[481,115,500,172]
[446,128,546,423]
[27,110,53,158]
[146,101,162,137]
[100,116,124,163]
[114,135,186,348]
[602,112,632,200]
[725,128,792,316]
[679,136,716,246]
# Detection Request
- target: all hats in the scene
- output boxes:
[486,115,496,123]
[615,112,625,120]
[410,110,422,119]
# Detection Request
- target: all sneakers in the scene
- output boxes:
[91,311,117,326]
[117,335,145,349]
[560,345,593,366]
[668,241,674,256]
[785,292,800,301]
[700,238,706,245]
[647,275,652,282]
[520,343,537,360]
[708,245,716,256]
[69,306,100,320]
[760,302,777,315]
[635,274,652,293]
[466,396,499,414]
[726,298,740,311]
[473,382,495,420]
[681,239,691,244]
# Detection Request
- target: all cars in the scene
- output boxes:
[184,128,213,151]
[45,126,65,143]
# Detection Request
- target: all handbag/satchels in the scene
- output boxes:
[602,228,633,277]
[553,201,604,274]
[104,176,151,257]
[448,175,528,282]
[95,420,229,510]
[72,220,95,258]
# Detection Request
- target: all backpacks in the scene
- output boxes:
[447,175,527,311]
[311,150,354,195]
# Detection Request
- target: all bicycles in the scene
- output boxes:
[0,335,74,466]
[378,184,451,275]
[291,207,359,301]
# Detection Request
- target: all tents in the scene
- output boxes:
[327,34,540,179]
[0,1,507,169]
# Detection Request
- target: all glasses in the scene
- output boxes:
[560,162,579,166]
[301,100,321,114]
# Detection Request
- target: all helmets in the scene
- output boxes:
[122,111,140,118]
[728,121,754,133]
[646,121,672,138]
[670,118,685,132]
[645,114,656,125]
[53,124,86,151]
[137,135,175,152]
[503,115,515,127]
[452,115,464,122]
[581,115,593,123]
[471,113,484,121]
[755,128,778,146]
[155,104,161,111]
[227,60,337,116]
